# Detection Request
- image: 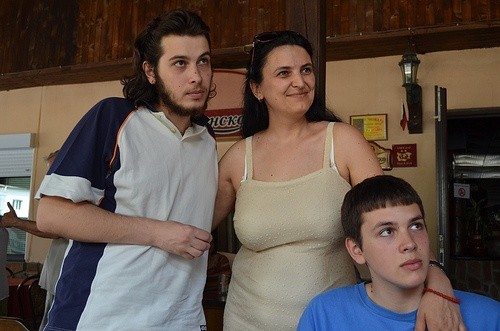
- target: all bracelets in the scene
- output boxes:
[424,256,449,272]
[423,287,460,304]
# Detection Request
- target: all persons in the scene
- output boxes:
[33,9,219,331]
[295,172,500,331]
[0,148,72,331]
[211,27,465,331]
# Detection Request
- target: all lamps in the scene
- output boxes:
[398,52,423,134]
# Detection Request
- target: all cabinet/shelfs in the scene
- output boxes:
[0,0,500,90]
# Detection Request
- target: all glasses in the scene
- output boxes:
[250,32,307,76]
